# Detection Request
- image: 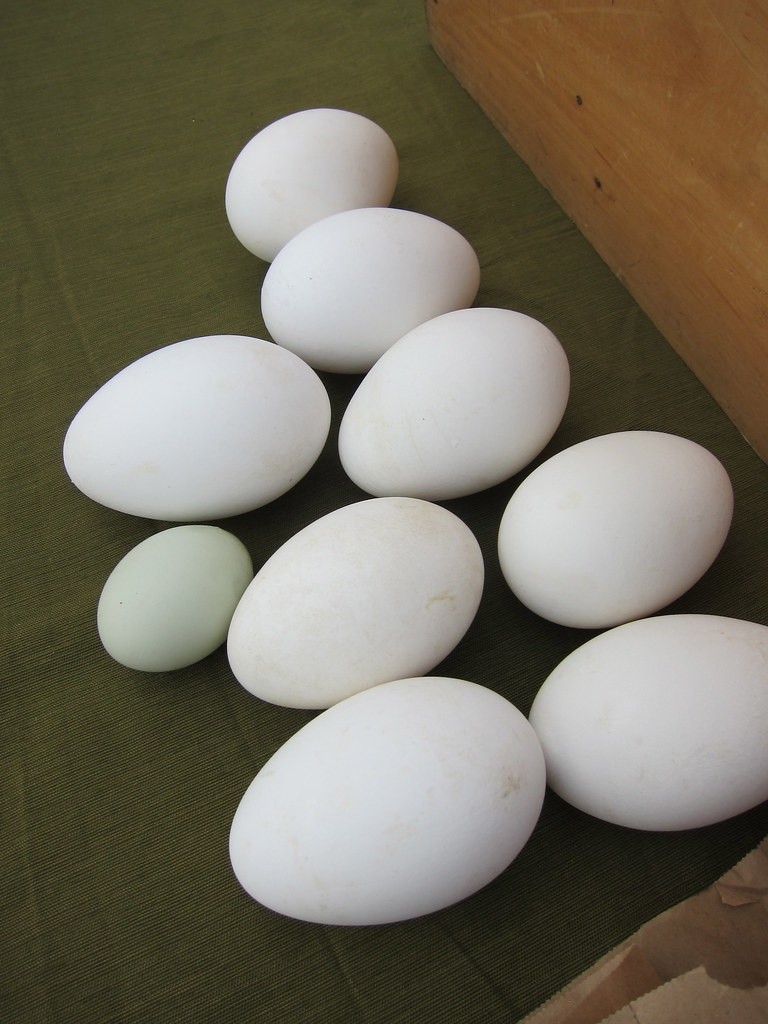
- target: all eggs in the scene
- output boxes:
[63,105,768,926]
